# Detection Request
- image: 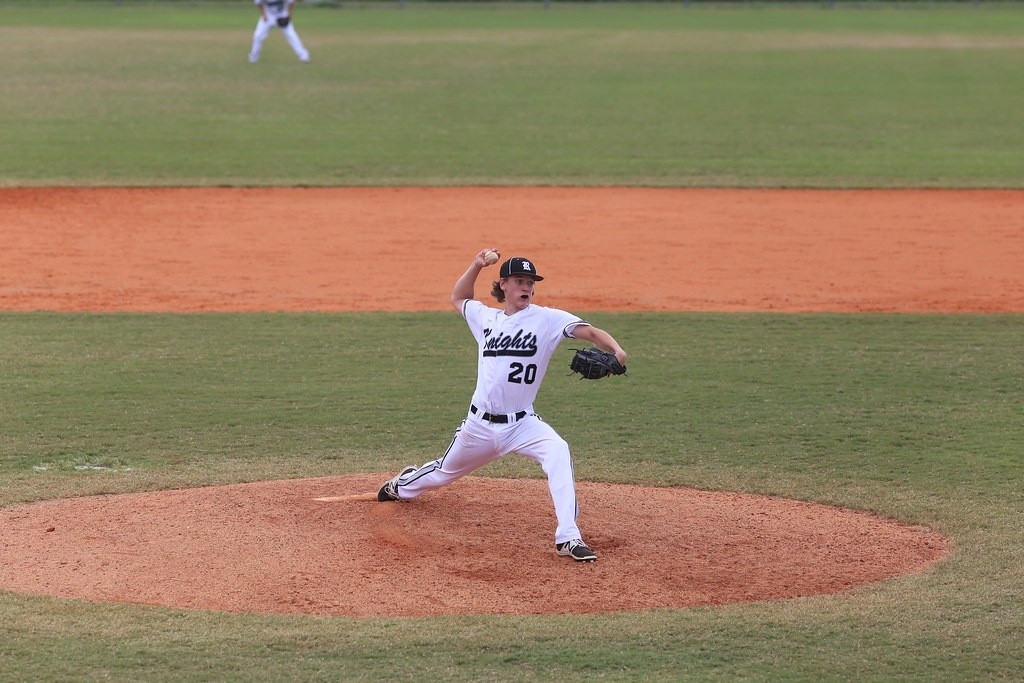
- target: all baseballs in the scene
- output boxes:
[484,251,499,264]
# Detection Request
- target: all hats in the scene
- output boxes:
[500,257,544,281]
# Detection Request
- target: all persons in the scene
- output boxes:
[378,245,626,561]
[246,0,309,65]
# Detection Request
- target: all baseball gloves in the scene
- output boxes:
[571,348,627,380]
[278,17,289,27]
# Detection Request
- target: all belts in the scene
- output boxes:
[471,404,526,423]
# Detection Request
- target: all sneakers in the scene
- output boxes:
[556,539,597,559]
[376,466,417,502]
